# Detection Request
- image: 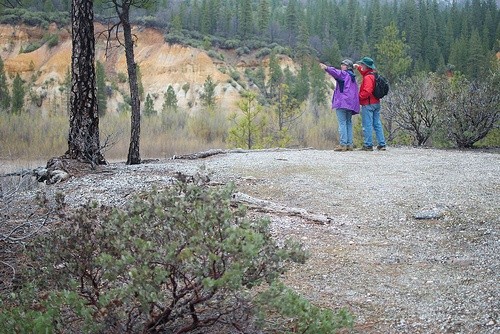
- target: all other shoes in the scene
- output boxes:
[334,145,347,151]
[377,145,386,150]
[359,146,373,151]
[346,146,353,151]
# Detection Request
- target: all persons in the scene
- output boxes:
[352,57,387,151]
[320,59,360,151]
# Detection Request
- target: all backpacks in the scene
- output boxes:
[366,71,389,98]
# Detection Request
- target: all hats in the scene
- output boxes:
[358,56,375,69]
[341,60,353,69]
